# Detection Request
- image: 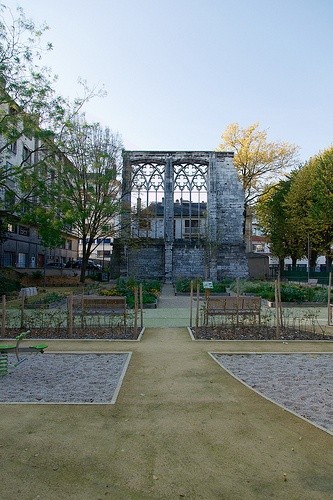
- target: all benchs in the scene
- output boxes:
[203,295,262,330]
[66,294,128,330]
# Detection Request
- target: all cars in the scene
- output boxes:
[72,260,102,269]
[66,261,76,268]
[46,262,67,268]
